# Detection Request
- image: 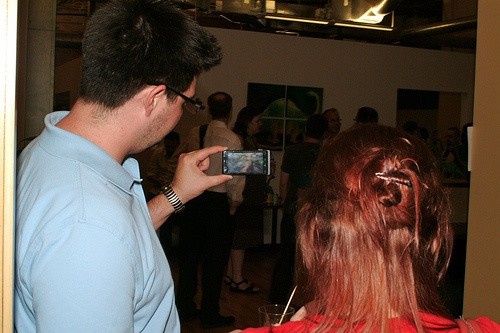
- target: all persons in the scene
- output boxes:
[12,0,232,333]
[268,115,324,305]
[352,106,379,125]
[224,106,264,293]
[165,92,246,328]
[145,128,181,201]
[241,123,500,332]
[322,109,343,157]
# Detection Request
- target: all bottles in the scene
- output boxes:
[267,183,273,205]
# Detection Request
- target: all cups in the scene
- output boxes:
[257,304,296,328]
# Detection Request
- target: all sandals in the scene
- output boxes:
[229,279,261,293]
[224,274,250,285]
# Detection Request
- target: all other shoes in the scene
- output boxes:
[199,314,236,329]
[181,304,200,320]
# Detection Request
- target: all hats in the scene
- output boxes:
[353,107,378,122]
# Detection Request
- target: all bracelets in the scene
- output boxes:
[161,185,186,214]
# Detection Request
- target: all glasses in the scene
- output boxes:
[328,117,341,122]
[147,79,205,115]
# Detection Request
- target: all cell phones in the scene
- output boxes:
[220,149,272,175]
